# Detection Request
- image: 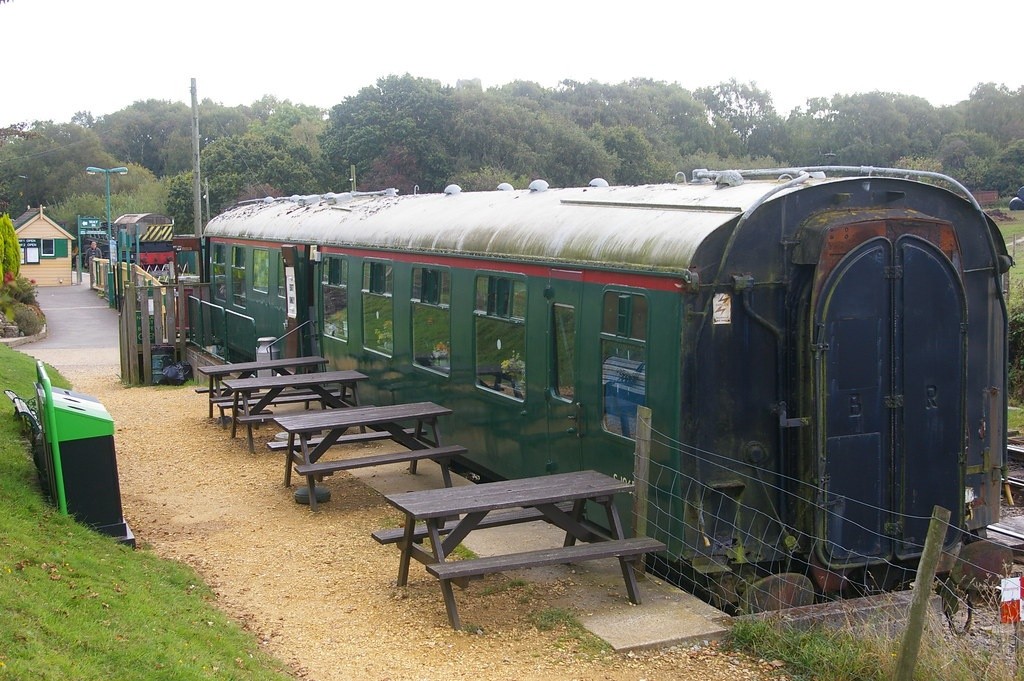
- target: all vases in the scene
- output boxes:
[384,341,393,351]
[510,376,522,398]
[436,359,449,367]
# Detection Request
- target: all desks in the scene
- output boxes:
[222,370,369,454]
[198,355,330,430]
[272,401,452,512]
[385,470,642,632]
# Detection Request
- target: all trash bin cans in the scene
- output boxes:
[256,337,280,378]
[33,361,136,551]
[151,346,176,385]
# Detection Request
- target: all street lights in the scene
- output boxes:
[85,166,129,308]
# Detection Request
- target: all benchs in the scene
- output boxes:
[372,500,587,546]
[426,536,666,580]
[195,386,228,393]
[217,392,352,408]
[294,444,468,476]
[235,405,376,424]
[209,386,338,403]
[267,428,427,451]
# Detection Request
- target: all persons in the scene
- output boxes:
[85,241,103,289]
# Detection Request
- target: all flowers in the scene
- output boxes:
[502,349,525,385]
[428,340,450,360]
[375,319,393,346]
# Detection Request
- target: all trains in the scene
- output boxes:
[188,162,1011,584]
[92,212,174,265]
